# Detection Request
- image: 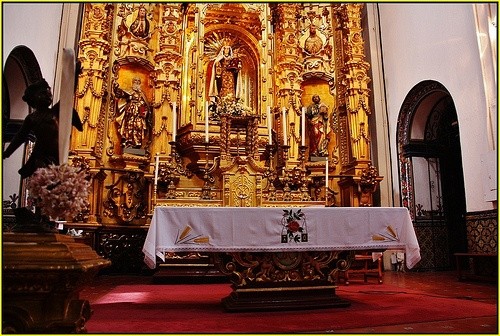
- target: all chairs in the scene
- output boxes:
[335,251,382,285]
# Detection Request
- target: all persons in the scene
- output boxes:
[304,24,323,53]
[119,77,151,149]
[130,7,149,36]
[4,78,82,219]
[307,94,330,157]
[215,44,240,102]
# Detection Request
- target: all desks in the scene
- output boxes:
[143,206,421,313]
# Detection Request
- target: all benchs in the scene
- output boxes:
[454,253,498,283]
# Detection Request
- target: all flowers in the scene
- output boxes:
[26,162,91,222]
[209,94,254,117]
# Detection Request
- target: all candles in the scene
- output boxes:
[282,107,287,145]
[155,156,159,185]
[172,101,176,142]
[326,157,329,187]
[205,101,209,142]
[267,106,274,144]
[302,107,305,146]
